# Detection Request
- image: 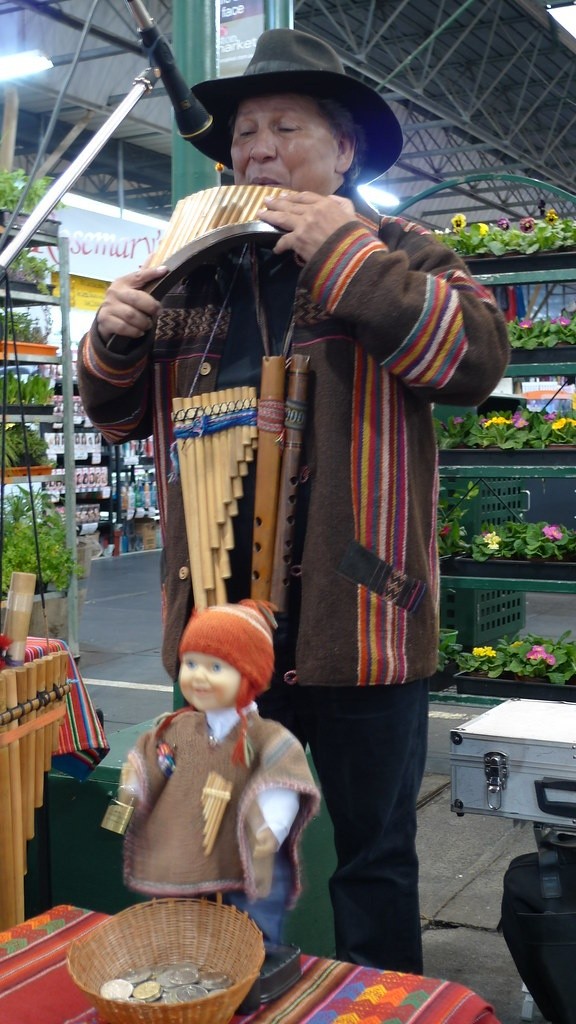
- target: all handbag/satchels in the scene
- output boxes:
[495,829,575,1024]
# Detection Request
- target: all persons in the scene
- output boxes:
[77,27,510,976]
[118,598,320,943]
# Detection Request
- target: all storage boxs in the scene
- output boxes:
[448,697,576,828]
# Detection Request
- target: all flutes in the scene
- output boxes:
[269,355,311,620]
[250,356,286,610]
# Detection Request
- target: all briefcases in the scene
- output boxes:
[447,696,576,829]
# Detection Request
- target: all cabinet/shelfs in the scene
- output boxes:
[0,227,82,664]
[387,174,576,708]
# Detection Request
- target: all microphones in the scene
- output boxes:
[123,0,214,141]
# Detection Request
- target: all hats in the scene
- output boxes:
[190,30,404,186]
